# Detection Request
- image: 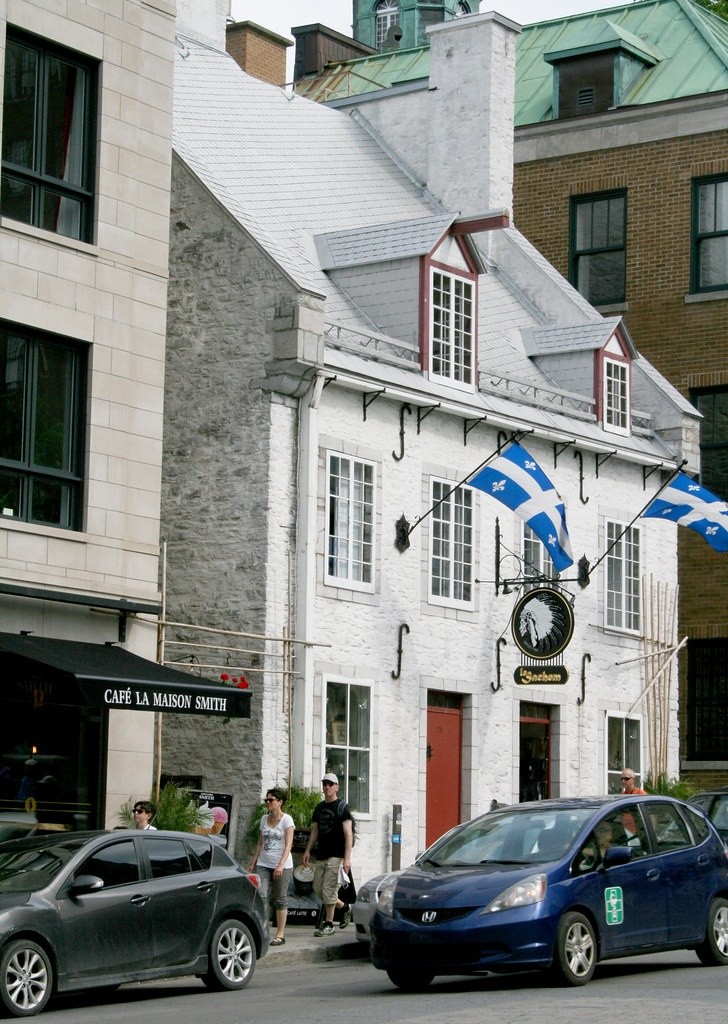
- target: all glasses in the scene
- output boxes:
[323,782,337,787]
[265,798,279,802]
[597,828,611,833]
[132,809,148,814]
[621,777,632,781]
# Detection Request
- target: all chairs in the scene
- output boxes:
[610,822,629,847]
[531,827,566,857]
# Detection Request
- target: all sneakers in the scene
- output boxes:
[314,925,336,937]
[339,903,352,929]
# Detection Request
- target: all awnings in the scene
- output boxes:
[0,632,253,720]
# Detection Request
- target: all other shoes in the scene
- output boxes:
[270,937,285,946]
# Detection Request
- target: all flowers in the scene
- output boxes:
[221,672,249,689]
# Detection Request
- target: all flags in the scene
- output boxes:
[640,470,728,552]
[467,443,574,573]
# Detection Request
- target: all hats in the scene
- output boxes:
[321,773,339,784]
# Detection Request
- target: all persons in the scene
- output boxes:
[132,801,158,830]
[595,822,613,857]
[247,788,297,946]
[302,772,354,937]
[621,768,656,846]
[18,759,62,802]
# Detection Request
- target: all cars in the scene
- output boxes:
[657,786,728,842]
[352,814,641,942]
[0,830,270,1016]
[370,795,728,994]
